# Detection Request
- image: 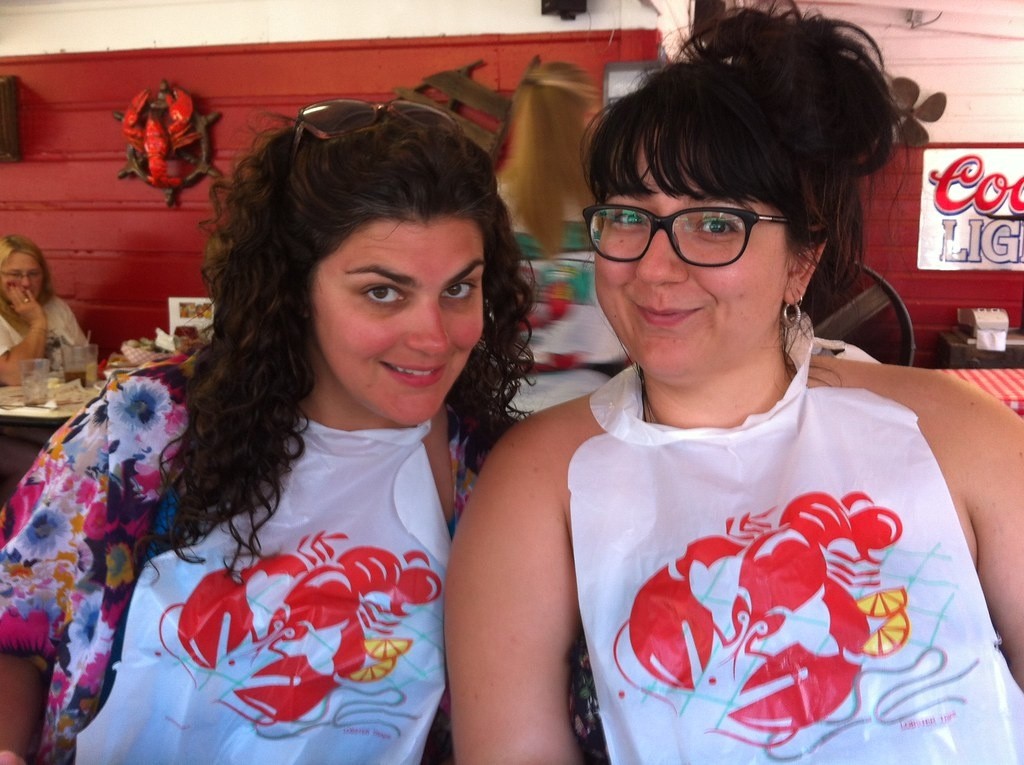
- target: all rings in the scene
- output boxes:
[24,298,28,303]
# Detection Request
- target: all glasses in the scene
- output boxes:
[0,270,42,281]
[582,203,788,267]
[289,97,457,162]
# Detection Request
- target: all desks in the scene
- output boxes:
[930,369,1023,420]
[0,387,100,425]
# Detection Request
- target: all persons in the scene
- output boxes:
[0,235,87,386]
[0,97,533,765]
[443,8,1024,765]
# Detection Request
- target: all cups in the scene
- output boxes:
[19,359,50,405]
[62,345,87,390]
[81,344,98,387]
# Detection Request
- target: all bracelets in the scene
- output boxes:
[29,327,46,335]
[645,403,656,423]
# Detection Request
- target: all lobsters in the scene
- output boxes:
[121,88,203,188]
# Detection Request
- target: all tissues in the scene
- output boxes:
[957,308,1009,352]
